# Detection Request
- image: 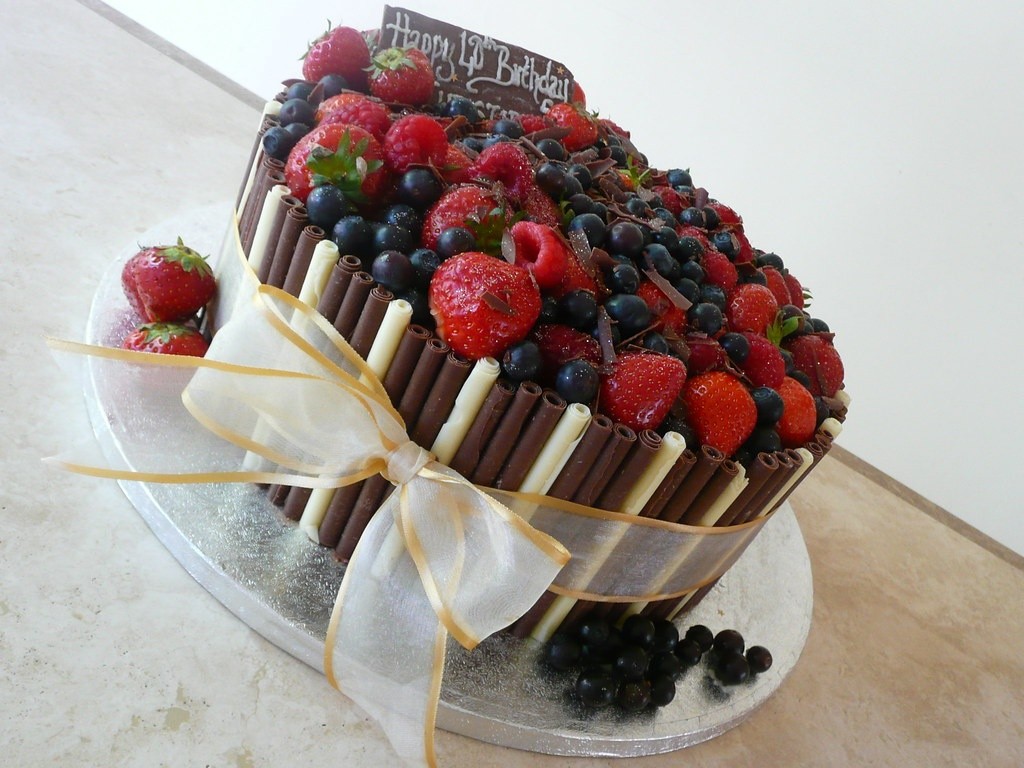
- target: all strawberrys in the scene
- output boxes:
[116,237,218,396]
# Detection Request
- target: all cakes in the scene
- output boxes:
[186,3,851,643]
